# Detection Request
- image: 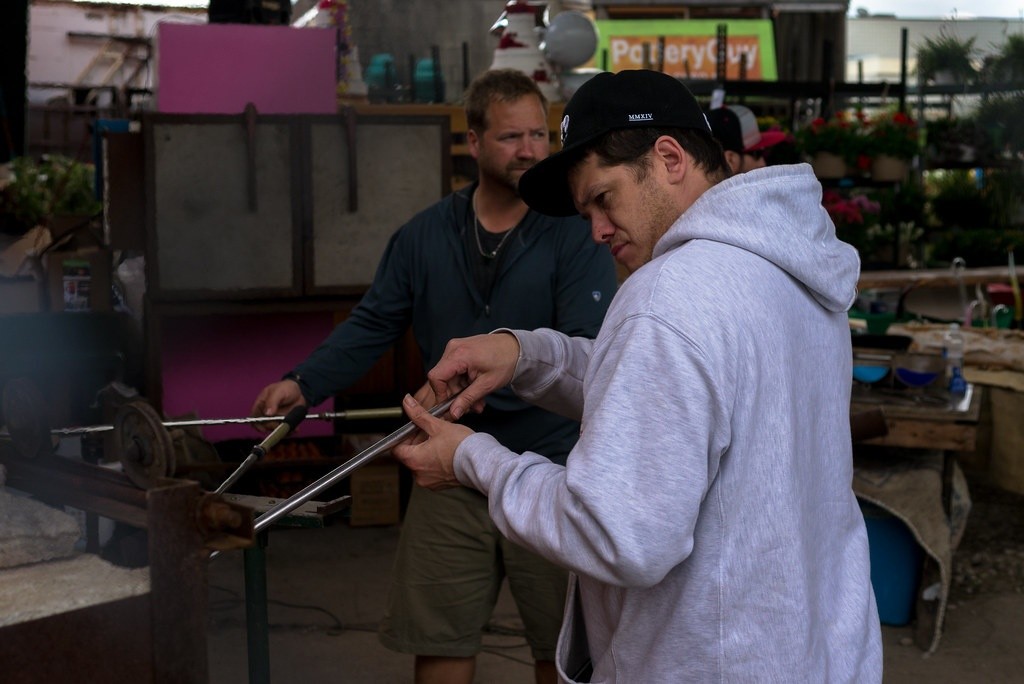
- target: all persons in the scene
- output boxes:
[706,105,787,176]
[250,67,617,683]
[394,69,884,684]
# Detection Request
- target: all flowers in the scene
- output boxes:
[751,108,923,248]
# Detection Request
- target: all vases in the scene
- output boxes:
[870,237,890,251]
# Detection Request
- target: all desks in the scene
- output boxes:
[848,382,985,663]
[856,264,1024,323]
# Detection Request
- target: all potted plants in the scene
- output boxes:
[867,187,900,228]
[982,56,1009,87]
[921,37,981,86]
[998,35,1024,82]
[923,90,1024,268]
[897,170,928,225]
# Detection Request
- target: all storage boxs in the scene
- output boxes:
[344,46,368,96]
[44,221,114,315]
[961,365,1024,495]
[340,456,400,527]
[153,21,339,117]
[157,318,336,440]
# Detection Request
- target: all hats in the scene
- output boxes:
[518,70,711,215]
[703,106,786,154]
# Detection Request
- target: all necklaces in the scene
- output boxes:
[473,188,528,257]
